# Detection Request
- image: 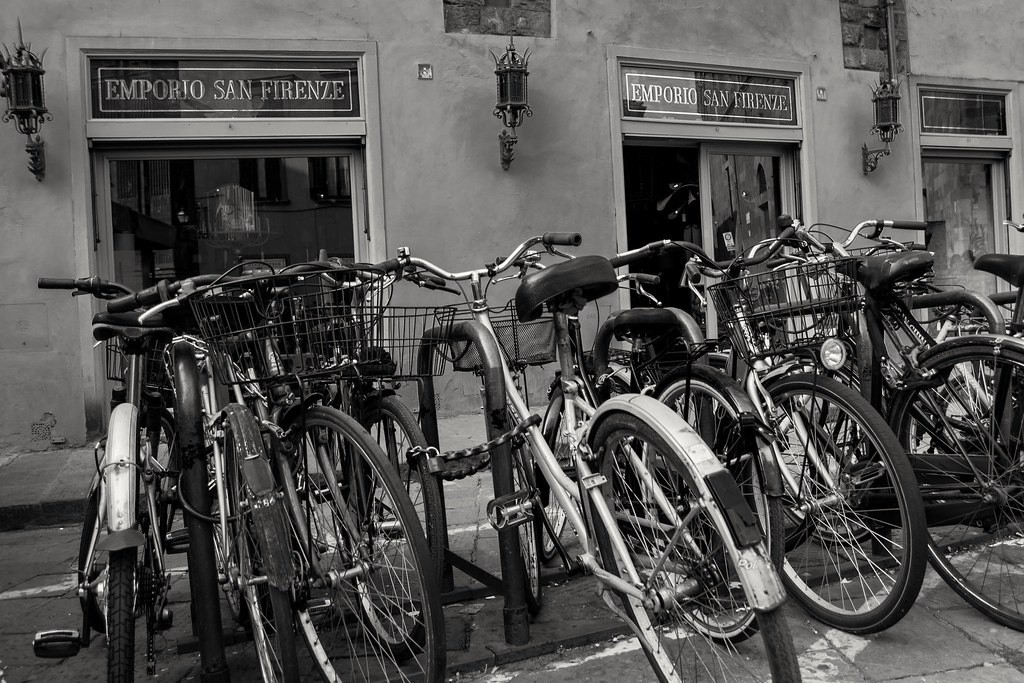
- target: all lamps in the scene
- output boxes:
[490,30,533,172]
[0,16,54,182]
[861,79,904,176]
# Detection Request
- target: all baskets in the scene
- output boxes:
[186,268,386,385]
[434,298,556,372]
[790,240,914,329]
[107,335,174,389]
[300,306,457,378]
[707,258,860,360]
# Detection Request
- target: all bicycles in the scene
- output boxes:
[28,214,1024,683]
